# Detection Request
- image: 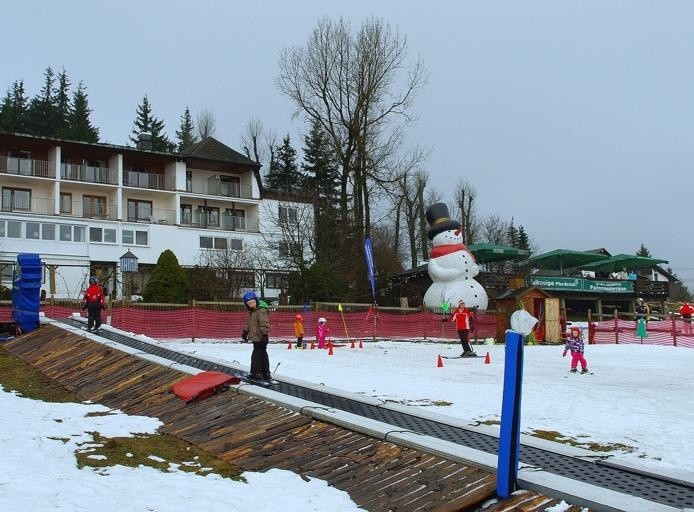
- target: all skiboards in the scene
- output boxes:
[81,326,105,336]
[441,356,486,359]
[234,372,280,386]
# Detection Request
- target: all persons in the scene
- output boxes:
[447,300,474,356]
[635,296,651,322]
[82,277,105,331]
[616,267,637,279]
[316,316,330,349]
[680,300,694,324]
[294,313,306,345]
[241,290,272,381]
[563,327,588,371]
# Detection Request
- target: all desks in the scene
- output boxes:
[157,219,168,224]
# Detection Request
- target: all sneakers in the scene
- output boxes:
[581,368,588,372]
[571,368,577,372]
[461,351,476,357]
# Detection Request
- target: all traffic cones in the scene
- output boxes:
[436,354,445,368]
[285,337,364,355]
[484,351,490,365]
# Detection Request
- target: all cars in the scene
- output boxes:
[122,291,145,303]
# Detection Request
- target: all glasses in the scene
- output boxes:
[460,302,465,305]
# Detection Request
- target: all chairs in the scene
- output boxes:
[148,214,158,224]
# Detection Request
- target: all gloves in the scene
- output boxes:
[242,329,248,342]
[262,334,268,345]
[563,351,566,357]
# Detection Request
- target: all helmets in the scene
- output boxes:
[89,275,99,284]
[243,291,259,305]
[295,314,303,321]
[318,318,326,323]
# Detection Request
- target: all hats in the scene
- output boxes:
[458,299,465,305]
[571,327,580,337]
